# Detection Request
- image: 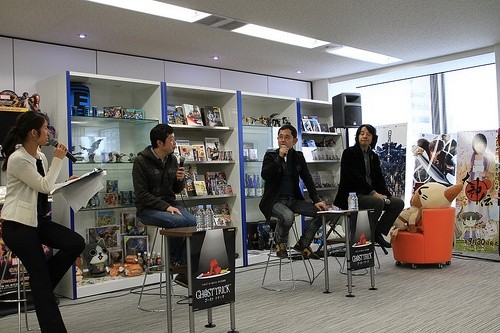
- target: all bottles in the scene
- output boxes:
[204,204,214,229]
[348,193,358,210]
[138,252,162,273]
[195,205,205,230]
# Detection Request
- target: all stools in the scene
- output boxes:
[0,246,41,332]
[339,209,385,276]
[258,214,314,292]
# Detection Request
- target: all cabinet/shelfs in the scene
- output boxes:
[297,97,356,254]
[240,91,299,267]
[162,82,247,280]
[33,71,171,299]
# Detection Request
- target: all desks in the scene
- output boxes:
[159,226,241,333]
[317,209,378,297]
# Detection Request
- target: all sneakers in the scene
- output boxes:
[275,242,289,259]
[293,241,319,259]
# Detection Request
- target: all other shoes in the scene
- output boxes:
[170,257,188,274]
[174,274,188,289]
[375,235,392,247]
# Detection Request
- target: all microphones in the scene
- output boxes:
[284,153,288,163]
[177,156,185,182]
[50,138,77,163]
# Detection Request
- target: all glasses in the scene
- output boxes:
[277,136,290,140]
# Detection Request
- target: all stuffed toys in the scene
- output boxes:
[84,238,108,277]
[390,182,464,243]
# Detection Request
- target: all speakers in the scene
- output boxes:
[332,93,363,129]
[316,128,389,254]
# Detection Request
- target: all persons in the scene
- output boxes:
[258,124,327,259]
[132,124,197,288]
[333,124,404,247]
[0,111,86,333]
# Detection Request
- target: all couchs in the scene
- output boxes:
[389,206,455,269]
